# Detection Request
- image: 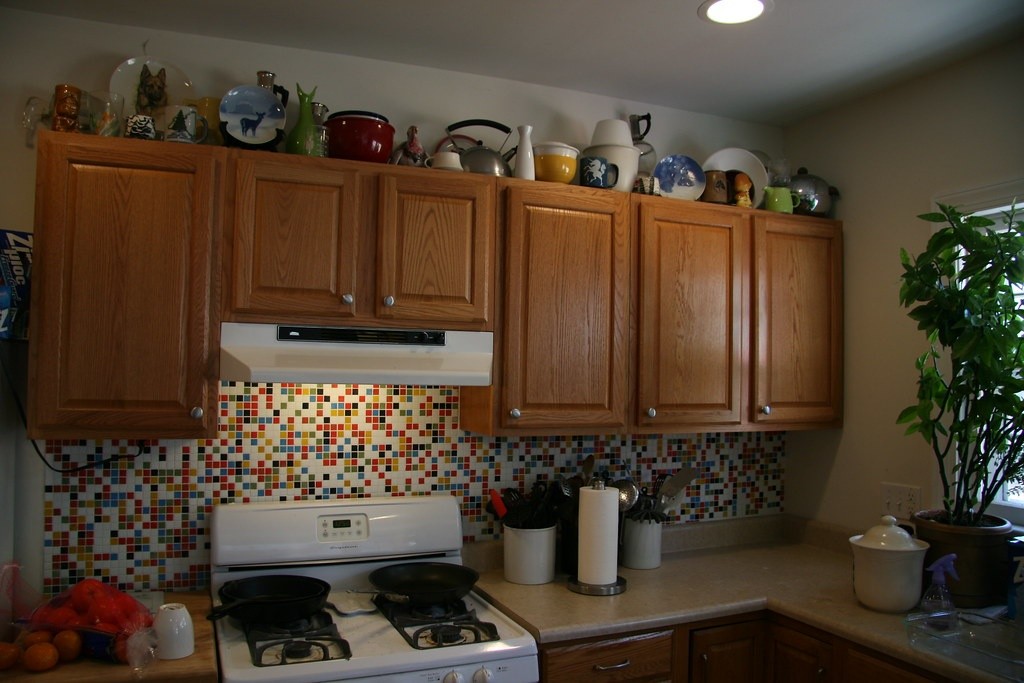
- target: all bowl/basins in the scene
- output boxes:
[323,110,396,163]
[533,141,581,184]
[583,144,642,192]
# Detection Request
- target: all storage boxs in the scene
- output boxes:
[0,228,33,341]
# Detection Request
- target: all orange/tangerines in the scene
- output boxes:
[0,630,82,671]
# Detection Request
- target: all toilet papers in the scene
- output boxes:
[578,486,618,586]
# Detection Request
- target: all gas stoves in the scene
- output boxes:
[212,495,537,683]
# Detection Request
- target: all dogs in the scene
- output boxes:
[135,63,168,116]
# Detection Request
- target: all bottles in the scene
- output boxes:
[285,82,324,156]
[254,70,277,94]
[514,125,536,181]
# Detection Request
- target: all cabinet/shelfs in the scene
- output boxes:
[460,172,745,436]
[750,206,843,432]
[223,147,498,331]
[540,609,771,683]
[26,129,229,439]
[768,608,957,683]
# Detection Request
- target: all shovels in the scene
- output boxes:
[582,454,595,486]
[649,470,693,512]
[504,487,526,513]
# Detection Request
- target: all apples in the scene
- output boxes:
[33,579,153,663]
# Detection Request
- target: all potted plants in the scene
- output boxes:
[895,196,1024,608]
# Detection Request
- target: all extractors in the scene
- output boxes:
[220,320,493,388]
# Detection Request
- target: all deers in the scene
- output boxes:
[240,111,264,137]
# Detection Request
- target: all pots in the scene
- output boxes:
[368,561,480,611]
[207,574,332,631]
[788,167,840,216]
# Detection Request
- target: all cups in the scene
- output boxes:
[424,152,464,172]
[622,518,662,570]
[502,524,558,586]
[163,105,208,145]
[153,602,195,660]
[125,115,157,140]
[86,89,125,137]
[704,170,728,203]
[849,534,930,614]
[304,124,329,158]
[580,157,619,189]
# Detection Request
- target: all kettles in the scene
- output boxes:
[445,119,519,177]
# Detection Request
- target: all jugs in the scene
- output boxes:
[762,185,801,214]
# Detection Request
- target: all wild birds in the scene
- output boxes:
[389,125,433,167]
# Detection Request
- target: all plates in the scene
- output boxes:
[701,148,769,209]
[218,84,287,144]
[653,153,706,201]
[109,56,193,132]
[434,134,478,154]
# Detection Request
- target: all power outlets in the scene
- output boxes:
[880,481,921,521]
